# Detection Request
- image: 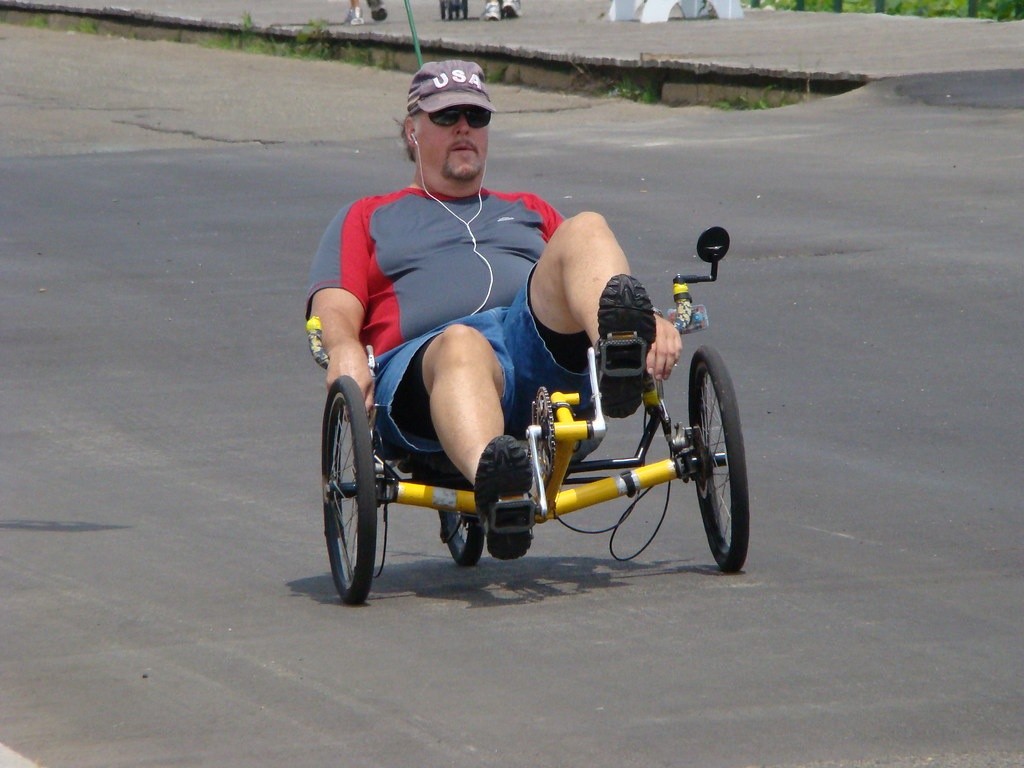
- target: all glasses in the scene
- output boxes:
[429,105,491,128]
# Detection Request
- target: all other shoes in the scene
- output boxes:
[345,7,365,25]
[481,2,501,21]
[475,435,534,560]
[367,0,388,21]
[597,274,656,418]
[501,0,522,17]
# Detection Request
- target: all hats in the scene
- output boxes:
[407,59,497,113]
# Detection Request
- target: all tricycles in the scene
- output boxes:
[306,223,755,607]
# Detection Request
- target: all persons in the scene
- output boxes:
[480,0,522,21]
[302,60,684,558]
[345,0,388,25]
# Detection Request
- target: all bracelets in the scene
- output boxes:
[651,305,663,318]
